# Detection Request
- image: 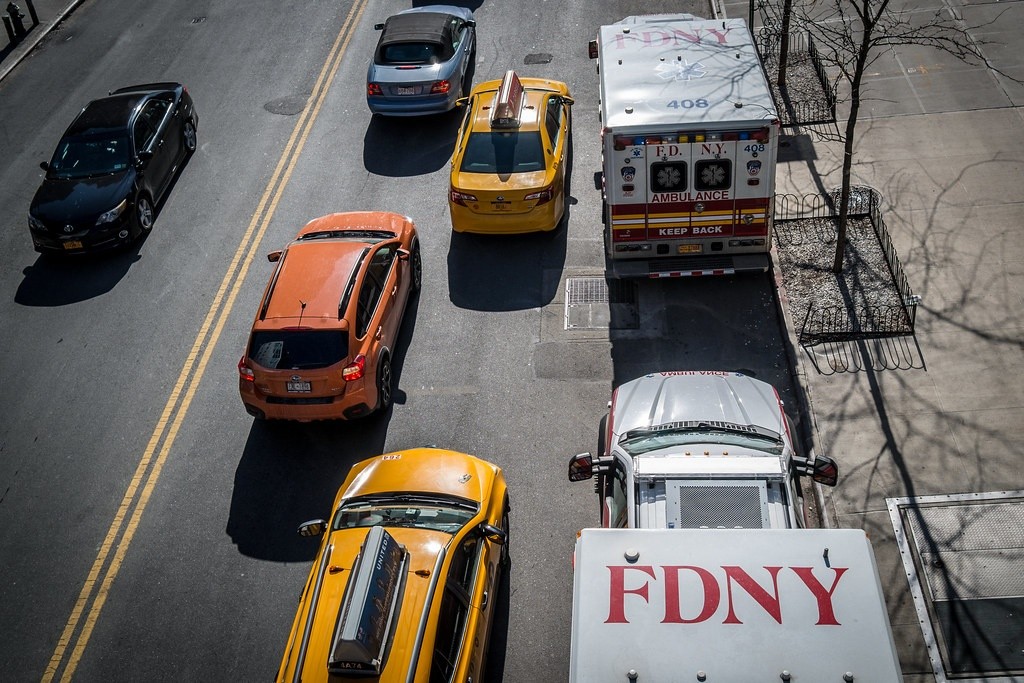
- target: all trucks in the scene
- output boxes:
[565,370,904,683]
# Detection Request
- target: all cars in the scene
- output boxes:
[27,81,199,255]
[271,444,512,683]
[365,5,476,116]
[237,212,423,427]
[447,69,575,235]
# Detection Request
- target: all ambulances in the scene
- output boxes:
[588,14,780,283]
